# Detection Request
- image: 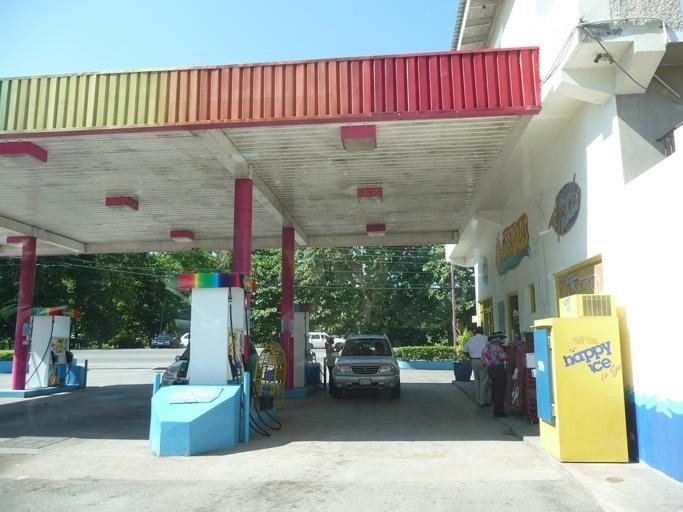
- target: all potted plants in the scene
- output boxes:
[452,326,479,384]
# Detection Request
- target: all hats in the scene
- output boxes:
[492,331,507,339]
[326,337,335,341]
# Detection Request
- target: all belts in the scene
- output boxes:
[471,357,481,359]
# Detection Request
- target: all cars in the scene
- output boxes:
[161,344,188,386]
[307,331,346,350]
[149,332,190,348]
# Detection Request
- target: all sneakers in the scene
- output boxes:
[476,399,508,418]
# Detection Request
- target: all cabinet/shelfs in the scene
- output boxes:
[505,344,538,428]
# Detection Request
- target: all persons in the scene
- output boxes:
[478,336,493,407]
[460,326,489,406]
[323,335,337,395]
[481,331,512,419]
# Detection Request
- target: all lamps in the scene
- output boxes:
[0,140,49,171]
[364,222,388,238]
[169,228,197,245]
[105,196,139,216]
[354,186,385,209]
[337,124,378,153]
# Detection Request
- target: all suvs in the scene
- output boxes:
[70,333,88,349]
[330,332,404,400]
